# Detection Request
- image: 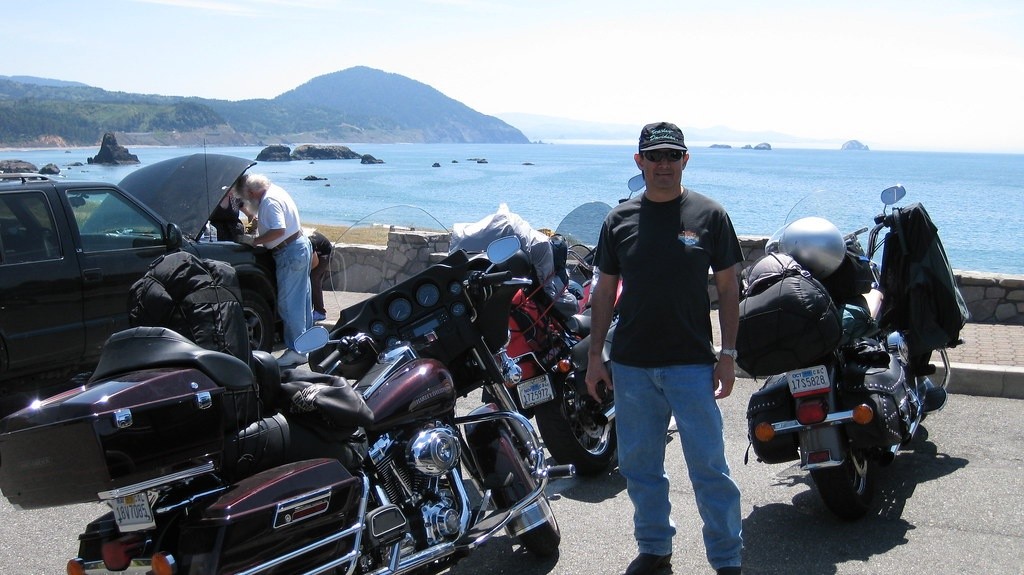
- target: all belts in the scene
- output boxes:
[272,229,303,253]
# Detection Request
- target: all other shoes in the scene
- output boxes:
[717,566,742,575]
[313,310,326,320]
[623,553,673,575]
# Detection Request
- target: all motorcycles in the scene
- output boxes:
[493,171,685,476]
[705,183,970,523]
[1,224,579,575]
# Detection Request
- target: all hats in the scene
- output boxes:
[639,122,688,151]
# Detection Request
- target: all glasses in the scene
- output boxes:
[643,151,684,162]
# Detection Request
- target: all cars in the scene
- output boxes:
[0,172,285,407]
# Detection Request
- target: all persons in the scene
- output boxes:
[584,121,745,575]
[209,173,333,368]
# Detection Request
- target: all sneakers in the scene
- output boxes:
[276,349,309,366]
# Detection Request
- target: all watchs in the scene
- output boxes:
[720,348,738,360]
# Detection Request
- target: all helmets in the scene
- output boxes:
[765,216,847,280]
[743,251,800,298]
